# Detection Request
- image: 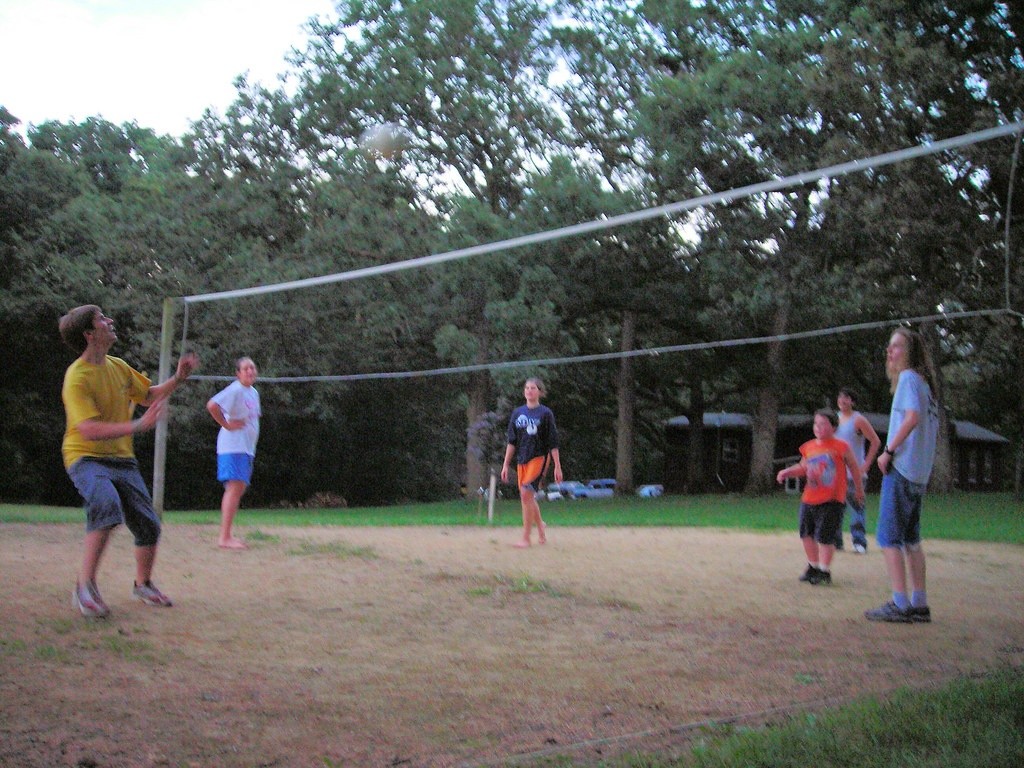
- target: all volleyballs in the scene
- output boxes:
[372,122,406,156]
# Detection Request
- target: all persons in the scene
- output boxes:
[832,388,881,554]
[207,356,262,548]
[59,304,201,616]
[863,326,939,624]
[501,378,563,548]
[777,407,864,585]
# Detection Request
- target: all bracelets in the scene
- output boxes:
[173,374,186,383]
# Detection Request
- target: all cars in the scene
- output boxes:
[585,479,616,497]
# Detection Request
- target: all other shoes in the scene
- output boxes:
[850,536,867,553]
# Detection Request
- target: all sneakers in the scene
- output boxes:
[131,579,172,608]
[904,605,933,622]
[799,563,815,580]
[863,600,913,623]
[70,579,110,618]
[809,570,831,583]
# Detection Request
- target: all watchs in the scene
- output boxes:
[885,444,895,455]
